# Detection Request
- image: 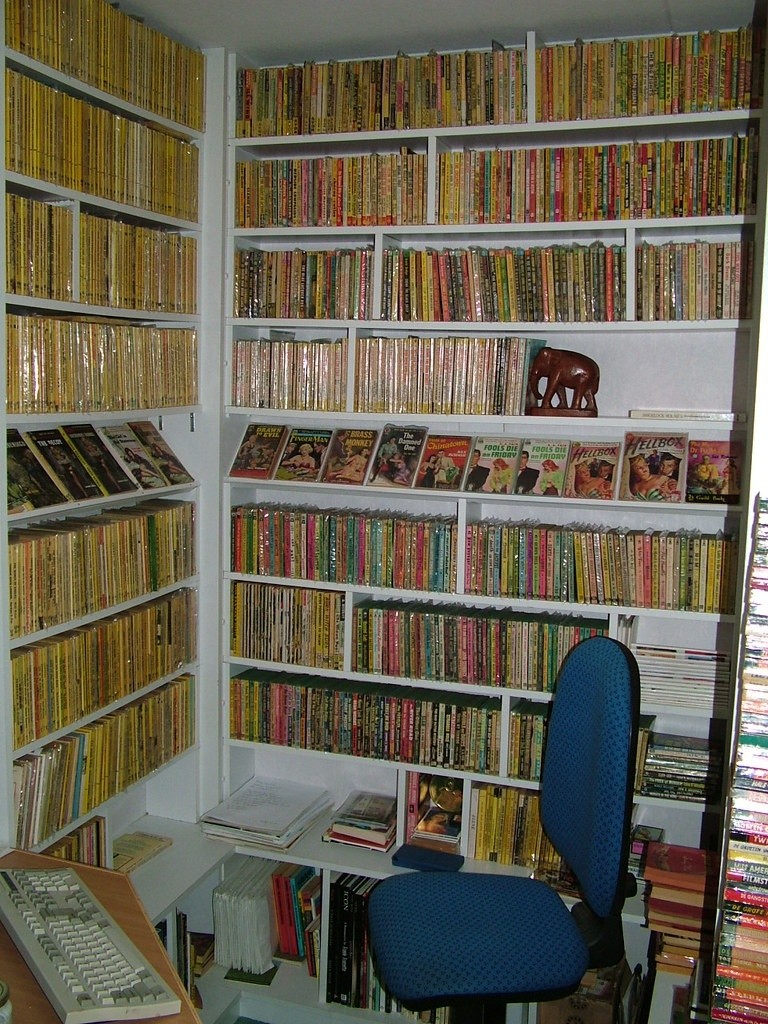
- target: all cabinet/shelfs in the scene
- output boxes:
[0,0,767,1024]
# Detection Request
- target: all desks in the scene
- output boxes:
[1,846,203,1023]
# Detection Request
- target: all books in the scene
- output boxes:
[0,0,768,1024]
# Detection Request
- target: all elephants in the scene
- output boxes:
[530,347,600,412]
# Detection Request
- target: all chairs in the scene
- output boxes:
[363,635,641,1023]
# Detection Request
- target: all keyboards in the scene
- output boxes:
[0,867,181,1024]
